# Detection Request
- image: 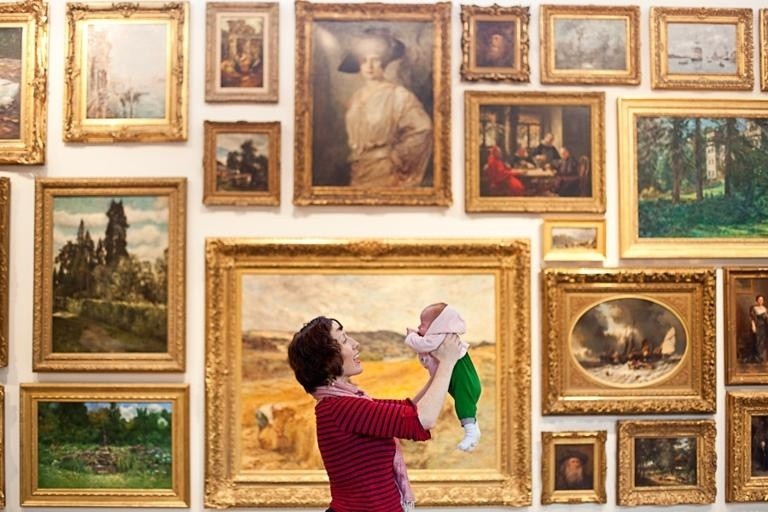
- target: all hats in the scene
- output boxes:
[482,28,510,44]
[336,26,405,76]
[559,449,587,461]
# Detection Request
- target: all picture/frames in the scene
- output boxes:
[204,237,533,510]
[616,96,768,260]
[464,89,607,215]
[292,0,455,209]
[0,0,48,167]
[460,3,531,83]
[33,175,187,374]
[725,390,768,504]
[722,264,768,386]
[616,418,717,506]
[19,382,190,509]
[648,6,754,91]
[542,430,607,505]
[541,266,717,416]
[203,120,283,207]
[539,4,641,86]
[0,386,6,512]
[0,178,10,368]
[65,1,188,144]
[544,218,608,263]
[205,1,281,104]
[760,8,768,92]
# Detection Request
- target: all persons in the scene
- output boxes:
[405,302,482,451]
[735,289,768,369]
[474,19,515,69]
[288,317,462,512]
[324,23,435,192]
[555,450,591,490]
[478,104,586,199]
